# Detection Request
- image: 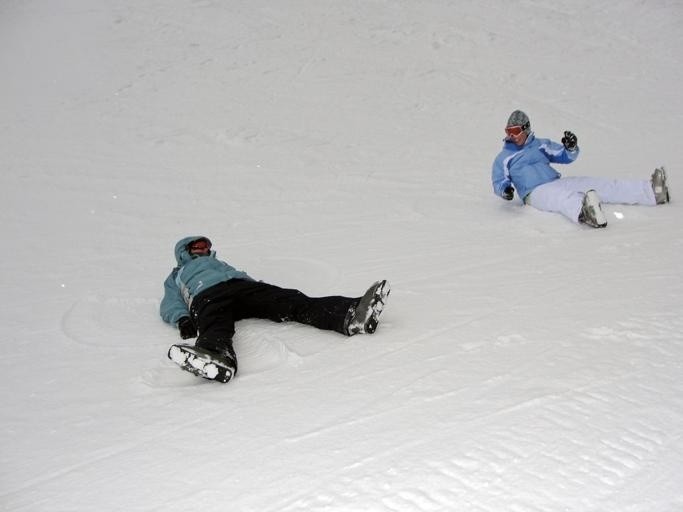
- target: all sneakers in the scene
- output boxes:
[652,169,667,205]
[582,191,606,228]
[175,345,236,380]
[347,283,379,333]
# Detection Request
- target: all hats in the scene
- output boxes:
[507,110,531,135]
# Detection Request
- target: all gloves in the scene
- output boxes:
[502,189,514,200]
[179,318,197,339]
[562,131,578,151]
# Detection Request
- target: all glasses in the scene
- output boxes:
[505,121,530,138]
[191,241,209,249]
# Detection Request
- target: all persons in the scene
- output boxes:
[491,110,670,228]
[160,236,390,384]
